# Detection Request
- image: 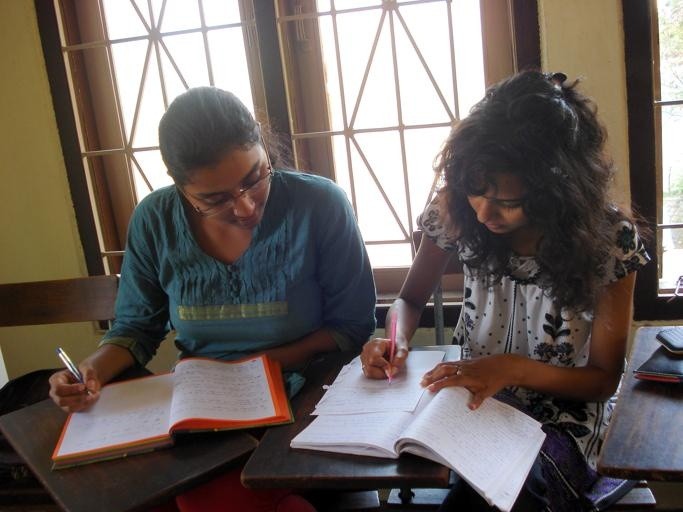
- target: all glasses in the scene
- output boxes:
[175,124,274,218]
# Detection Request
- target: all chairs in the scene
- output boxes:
[0,364,154,509]
[412,231,465,346]
[567,475,633,512]
[1,272,124,512]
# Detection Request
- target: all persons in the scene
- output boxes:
[360,70,637,509]
[48,85,380,508]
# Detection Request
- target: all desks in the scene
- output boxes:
[240,345,461,512]
[1,365,258,512]
[594,326,681,512]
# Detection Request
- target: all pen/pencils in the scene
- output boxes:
[388,311,397,386]
[56,345,94,398]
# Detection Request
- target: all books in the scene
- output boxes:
[50,355,294,470]
[288,385,550,510]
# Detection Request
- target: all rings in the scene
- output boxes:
[456,366,462,375]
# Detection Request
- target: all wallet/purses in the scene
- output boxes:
[632,336,683,390]
